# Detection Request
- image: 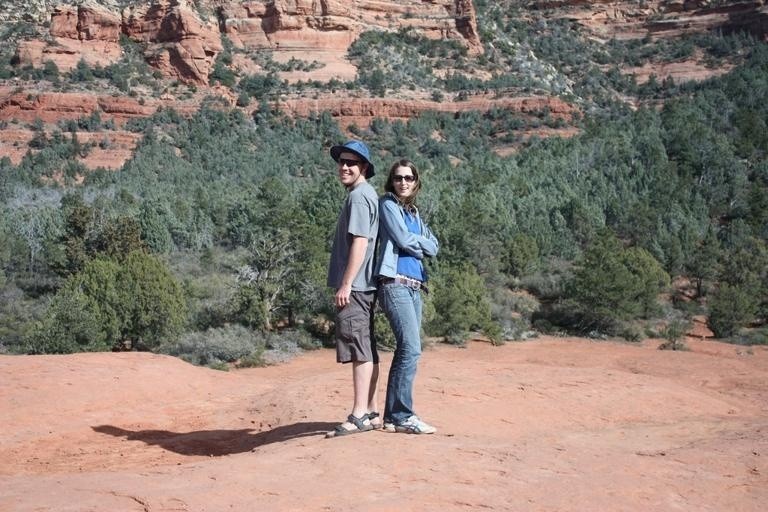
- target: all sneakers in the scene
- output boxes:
[385,416,436,434]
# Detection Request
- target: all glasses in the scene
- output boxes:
[393,175,414,182]
[339,158,359,167]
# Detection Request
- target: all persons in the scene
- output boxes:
[371,158,440,435]
[325,138,384,440]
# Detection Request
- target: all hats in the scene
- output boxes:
[330,141,375,179]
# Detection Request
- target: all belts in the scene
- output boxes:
[378,276,429,295]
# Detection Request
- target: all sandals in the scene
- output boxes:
[325,411,382,438]
